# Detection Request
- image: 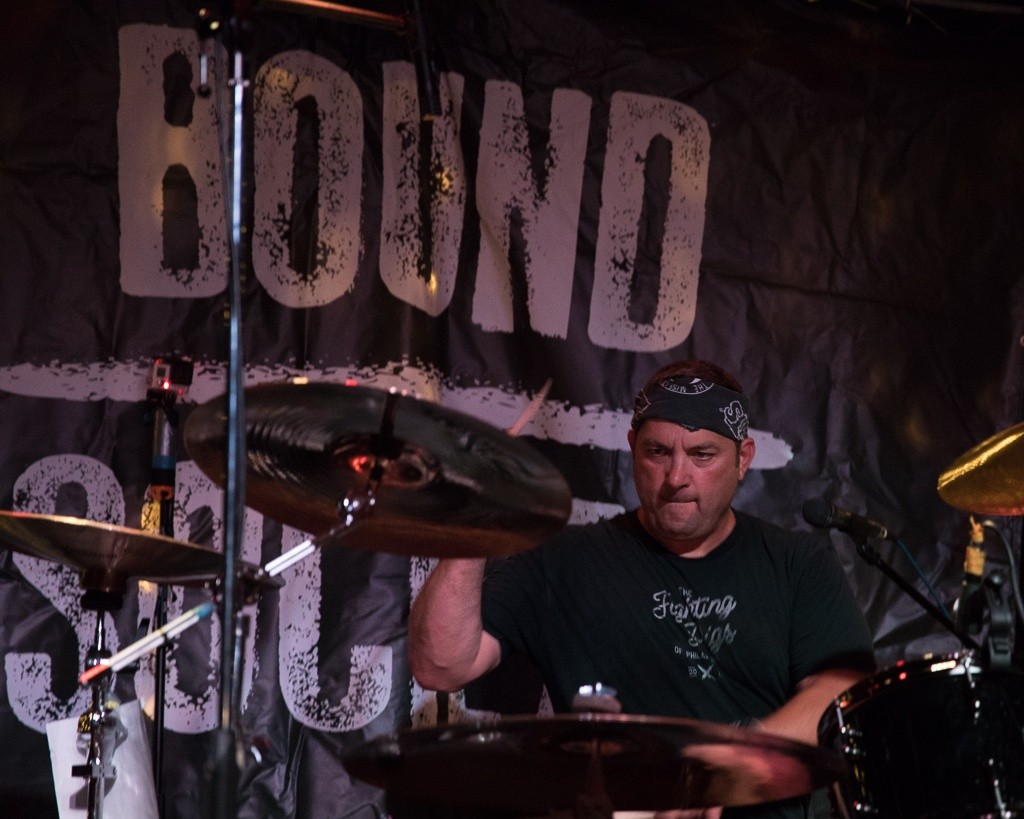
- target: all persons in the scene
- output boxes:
[407,362,877,819]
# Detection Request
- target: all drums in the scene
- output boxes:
[815,652,1024,819]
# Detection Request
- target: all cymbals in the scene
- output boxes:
[183,380,573,560]
[935,421,1024,517]
[337,716,841,812]
[0,508,287,594]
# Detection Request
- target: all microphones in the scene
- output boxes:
[147,355,194,500]
[953,524,987,636]
[802,497,898,543]
